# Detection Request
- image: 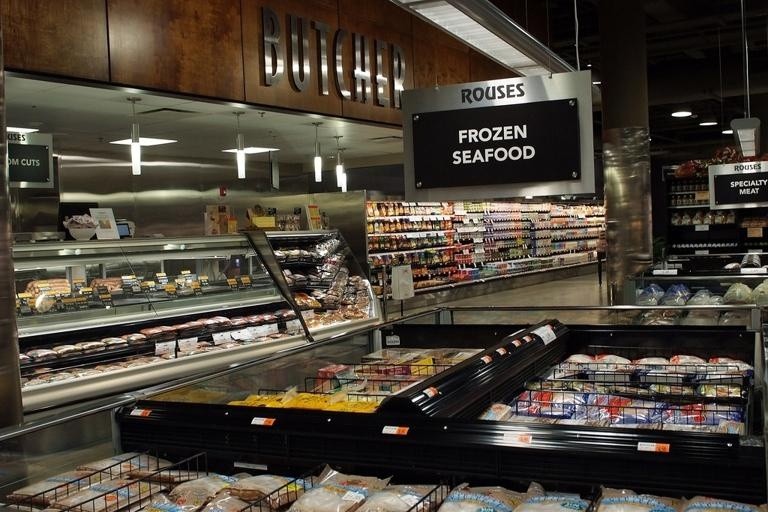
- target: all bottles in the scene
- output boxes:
[227,216,238,234]
[266,207,302,231]
[320,210,329,230]
[218,206,227,234]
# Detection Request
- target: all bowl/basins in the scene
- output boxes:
[68,228,97,240]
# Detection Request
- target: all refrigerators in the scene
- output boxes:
[0,305,768,511]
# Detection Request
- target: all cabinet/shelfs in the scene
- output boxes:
[1,231,310,455]
[257,189,606,316]
[265,227,385,383]
[651,141,768,270]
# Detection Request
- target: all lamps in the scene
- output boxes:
[232,111,247,179]
[333,135,342,186]
[339,148,348,192]
[728,1,761,159]
[387,0,602,108]
[129,97,143,175]
[311,121,323,182]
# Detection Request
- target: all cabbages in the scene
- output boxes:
[724,283,752,305]
[752,279,768,304]
